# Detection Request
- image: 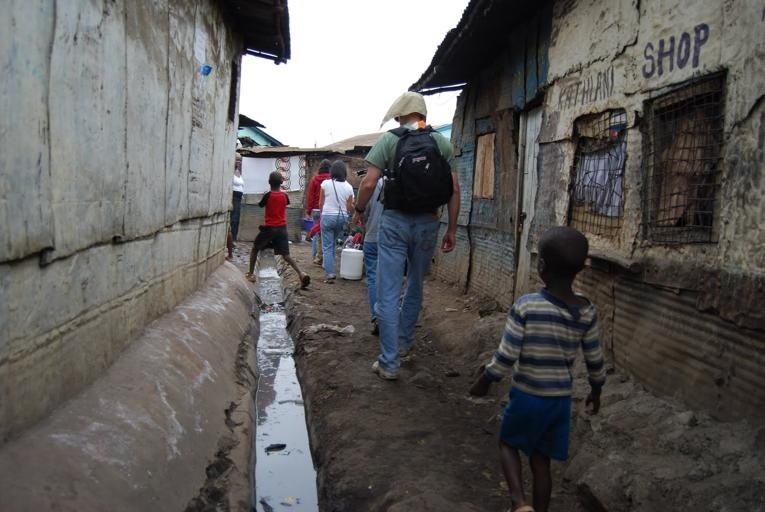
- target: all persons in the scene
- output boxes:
[351,92,460,378]
[362,173,387,334]
[468,226,606,511]
[245,171,311,288]
[319,160,355,284]
[230,151,246,245]
[306,159,332,260]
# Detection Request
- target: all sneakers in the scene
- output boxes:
[312,256,336,285]
[245,273,256,282]
[300,272,310,289]
[369,320,380,336]
[399,352,411,362]
[371,360,400,381]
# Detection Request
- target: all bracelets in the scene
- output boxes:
[355,204,366,213]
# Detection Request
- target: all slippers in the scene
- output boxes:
[225,255,236,262]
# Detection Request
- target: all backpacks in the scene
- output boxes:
[384,124,452,210]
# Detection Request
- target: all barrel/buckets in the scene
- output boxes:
[339,248,364,281]
